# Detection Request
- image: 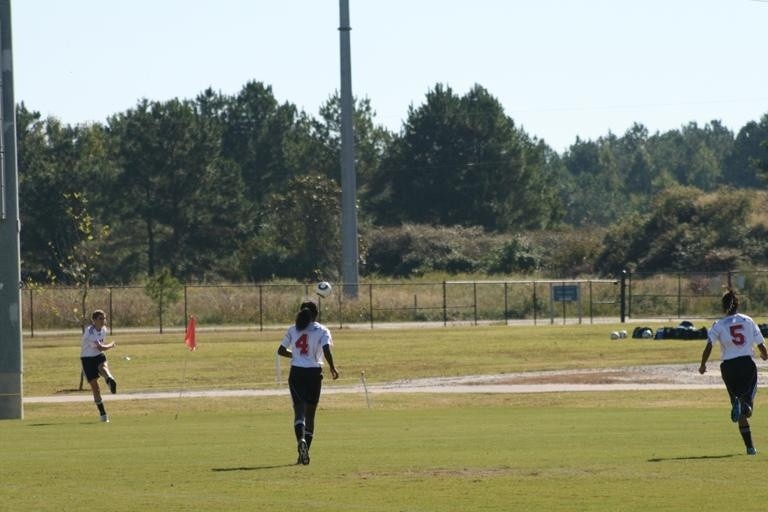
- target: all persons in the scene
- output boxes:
[278,300,340,465]
[698,284,768,454]
[79,309,116,423]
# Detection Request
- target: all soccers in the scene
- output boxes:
[316,282,332,298]
[642,329,652,339]
[610,330,620,340]
[619,330,627,339]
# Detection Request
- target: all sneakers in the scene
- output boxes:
[99,414,108,423]
[110,378,116,393]
[731,395,741,421]
[297,440,310,465]
